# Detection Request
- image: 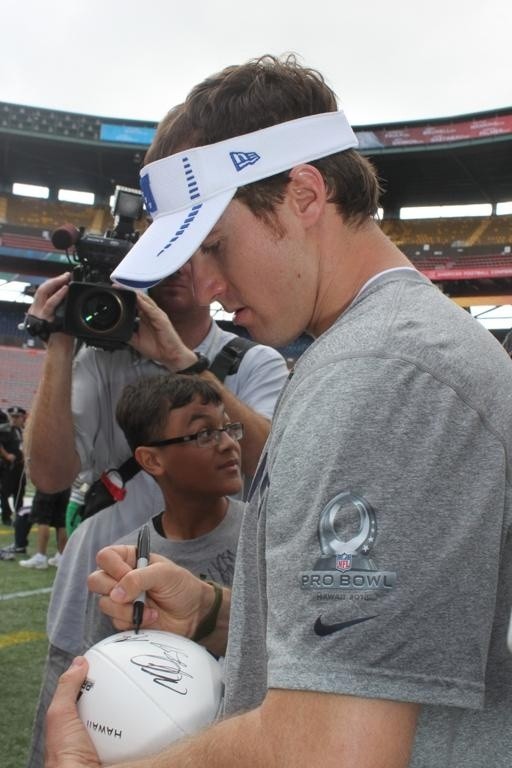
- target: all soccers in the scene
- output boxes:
[77,629,223,765]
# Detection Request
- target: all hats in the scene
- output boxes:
[110,108,360,292]
[6,405,26,417]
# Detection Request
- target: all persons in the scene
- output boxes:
[84,375,253,649]
[27,254,295,767]
[45,53,512,767]
[17,485,69,569]
[63,465,96,535]
[0,404,28,526]
[1,491,36,554]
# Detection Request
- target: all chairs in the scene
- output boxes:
[373,215,512,269]
[0,192,153,257]
[0,300,86,352]
[216,320,317,360]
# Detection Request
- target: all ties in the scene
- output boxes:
[15,425,22,440]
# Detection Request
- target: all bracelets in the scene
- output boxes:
[190,579,224,644]
[175,350,211,375]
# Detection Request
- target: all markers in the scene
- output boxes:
[132,524,150,635]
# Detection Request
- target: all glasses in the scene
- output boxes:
[142,422,245,449]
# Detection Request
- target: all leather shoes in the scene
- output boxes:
[2,516,15,526]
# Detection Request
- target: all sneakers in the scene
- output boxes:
[1,543,27,555]
[18,553,48,569]
[47,550,62,567]
[0,548,13,560]
